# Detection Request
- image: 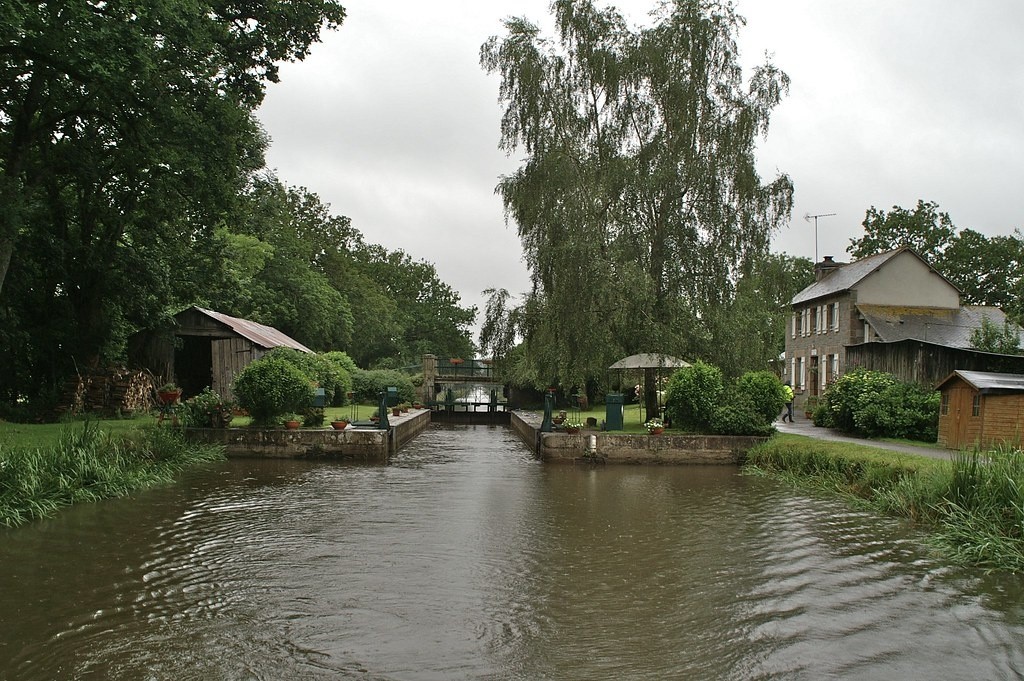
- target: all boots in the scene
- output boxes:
[782,414,795,423]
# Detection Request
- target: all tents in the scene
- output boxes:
[606,353,693,425]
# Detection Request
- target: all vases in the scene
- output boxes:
[649,427,663,435]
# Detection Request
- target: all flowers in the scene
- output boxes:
[643,417,663,433]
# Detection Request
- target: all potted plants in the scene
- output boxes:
[331,415,351,430]
[282,414,301,429]
[804,405,814,419]
[158,383,183,427]
[369,400,422,422]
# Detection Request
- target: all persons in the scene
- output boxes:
[782,378,796,424]
[664,395,673,428]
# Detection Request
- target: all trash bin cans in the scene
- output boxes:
[384,385,397,407]
[307,387,325,425]
[604,394,625,431]
